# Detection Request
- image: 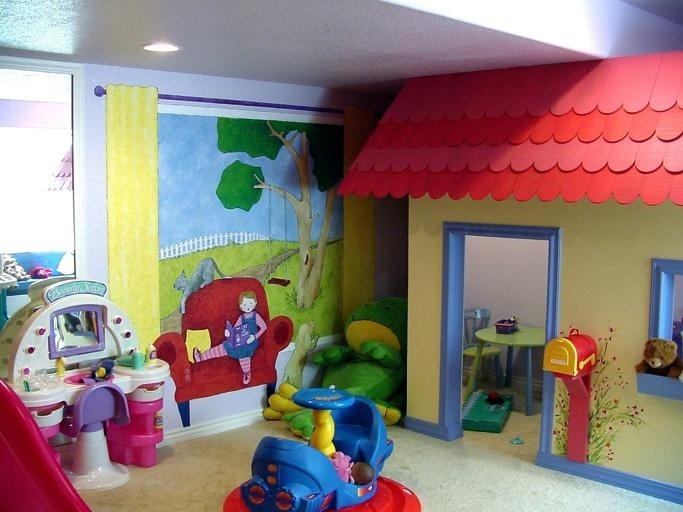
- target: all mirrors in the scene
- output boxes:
[0,55,88,325]
[49,305,107,359]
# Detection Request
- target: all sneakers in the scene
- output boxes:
[192,348,202,365]
[242,372,252,386]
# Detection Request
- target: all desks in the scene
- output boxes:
[464,325,546,416]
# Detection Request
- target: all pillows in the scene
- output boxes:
[56,251,74,274]
[185,329,211,363]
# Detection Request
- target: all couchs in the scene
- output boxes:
[0,250,76,296]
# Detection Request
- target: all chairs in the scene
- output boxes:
[60,382,130,491]
[462,308,502,386]
[155,276,294,427]
